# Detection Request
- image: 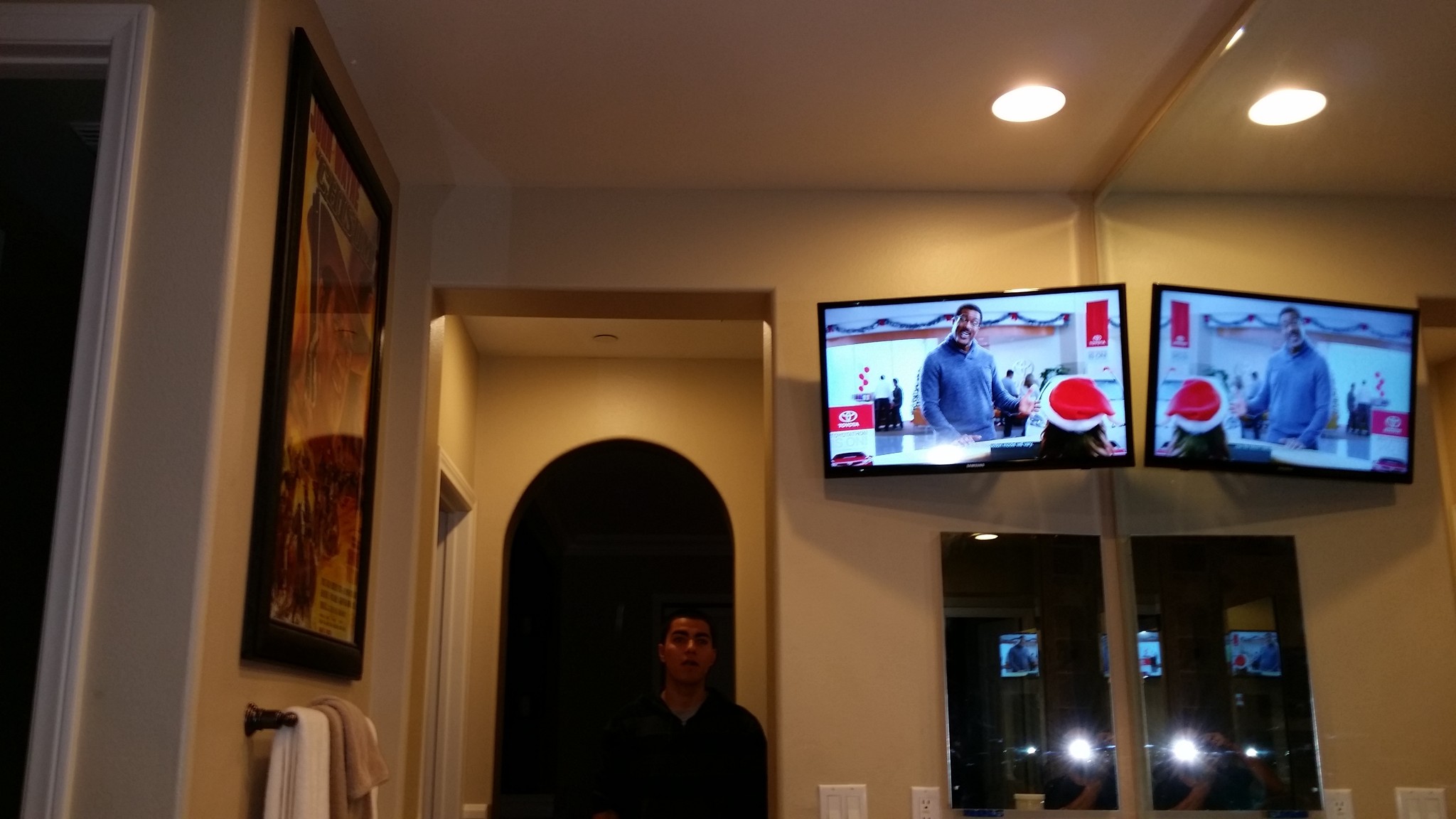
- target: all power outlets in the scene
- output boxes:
[1319,788,1355,819]
[911,785,943,819]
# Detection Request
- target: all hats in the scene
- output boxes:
[1232,653,1249,669]
[1040,374,1115,435]
[1158,376,1227,434]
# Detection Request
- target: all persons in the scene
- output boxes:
[1227,306,1333,451]
[1345,382,1357,432]
[1353,378,1371,436]
[997,369,1019,426]
[593,607,770,819]
[1165,378,1230,460]
[920,303,1042,447]
[1151,727,1285,811]
[1213,370,1242,438]
[1043,727,1120,811]
[1036,374,1118,458]
[1247,371,1265,439]
[1020,373,1040,436]
[892,378,904,429]
[873,375,892,431]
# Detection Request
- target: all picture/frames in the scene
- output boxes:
[239,22,394,680]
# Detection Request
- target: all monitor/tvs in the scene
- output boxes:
[1145,282,1419,486]
[815,282,1135,480]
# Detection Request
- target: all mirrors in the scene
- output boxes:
[1086,1,1455,819]
